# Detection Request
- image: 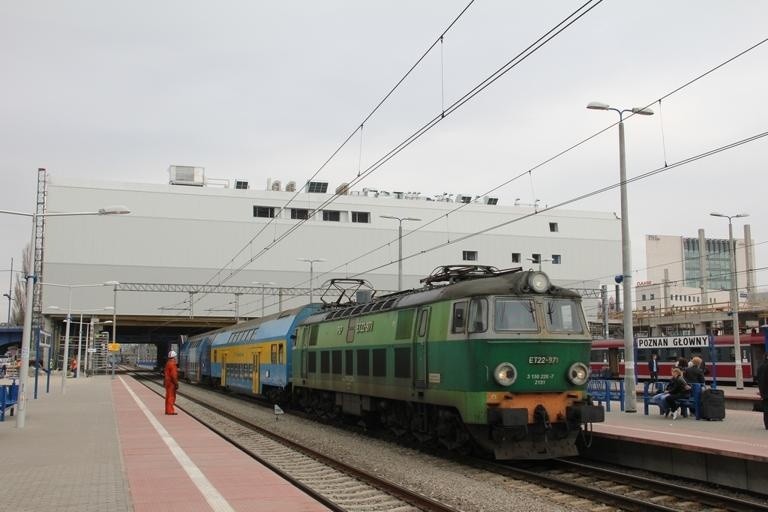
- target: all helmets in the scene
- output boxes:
[168,350,177,358]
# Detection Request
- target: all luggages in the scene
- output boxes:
[701,389,725,421]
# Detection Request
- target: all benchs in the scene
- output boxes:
[0,385,18,421]
[587,380,705,420]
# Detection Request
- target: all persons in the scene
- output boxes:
[164,350,179,415]
[757,351,768,430]
[647,353,659,388]
[69,356,77,378]
[653,355,708,420]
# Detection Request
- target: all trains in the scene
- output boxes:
[590,334,766,385]
[177,266,604,462]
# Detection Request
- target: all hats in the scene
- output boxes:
[672,368,681,375]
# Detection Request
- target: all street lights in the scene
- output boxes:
[0,208,131,428]
[527,255,554,271]
[586,101,655,411]
[252,282,274,317]
[381,215,421,290]
[710,213,750,389]
[300,259,326,304]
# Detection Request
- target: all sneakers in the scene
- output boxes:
[666,409,670,416]
[673,407,681,419]
